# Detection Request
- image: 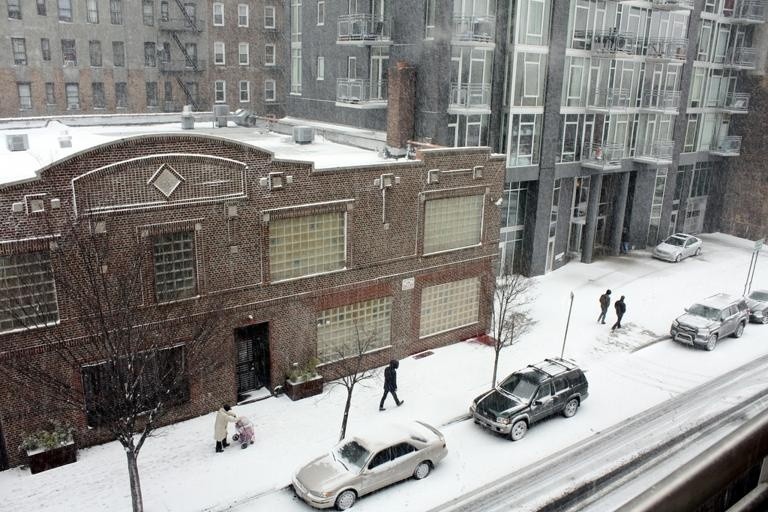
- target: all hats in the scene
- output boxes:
[224,405,231,410]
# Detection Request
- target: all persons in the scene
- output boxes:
[379,358,405,411]
[621,226,630,254]
[598,289,612,325]
[213,403,240,453]
[611,295,626,331]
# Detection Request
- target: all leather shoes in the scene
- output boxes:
[398,400,404,406]
[217,443,230,452]
[380,409,386,411]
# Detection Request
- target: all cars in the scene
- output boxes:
[741,290,768,324]
[651,232,703,263]
[292,418,448,512]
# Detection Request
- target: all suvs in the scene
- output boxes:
[469,357,589,441]
[670,293,750,351]
